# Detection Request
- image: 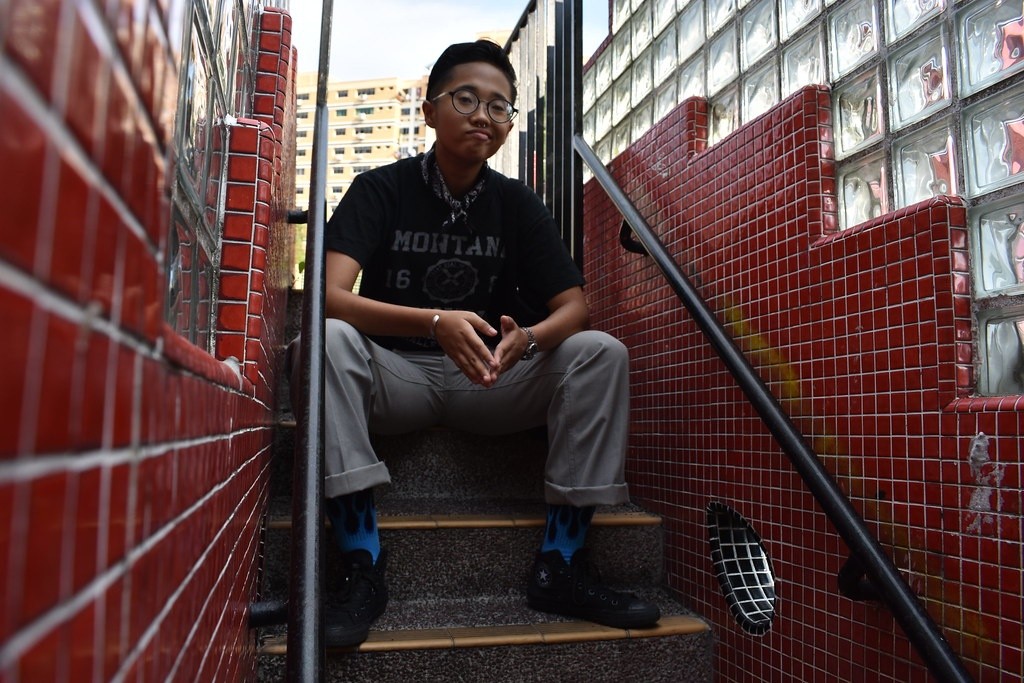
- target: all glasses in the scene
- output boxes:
[431,88,519,123]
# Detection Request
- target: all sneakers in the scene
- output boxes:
[525,547,661,630]
[320,549,389,649]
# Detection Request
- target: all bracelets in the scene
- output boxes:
[430,314,439,339]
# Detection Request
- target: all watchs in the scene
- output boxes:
[521,327,537,361]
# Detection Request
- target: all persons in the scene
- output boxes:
[286,41,663,648]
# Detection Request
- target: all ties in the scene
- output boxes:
[421,140,490,235]
